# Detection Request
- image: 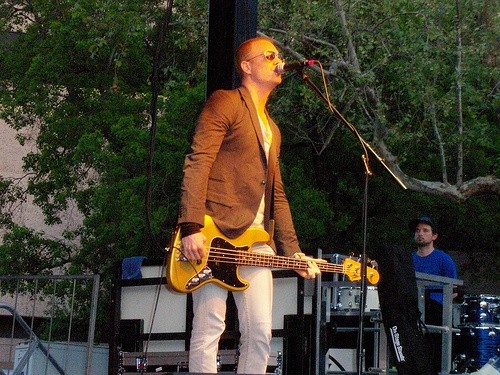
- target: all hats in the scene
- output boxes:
[409,216,436,233]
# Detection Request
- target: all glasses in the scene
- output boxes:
[246,50,283,63]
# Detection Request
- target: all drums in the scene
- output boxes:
[454,292,499,375]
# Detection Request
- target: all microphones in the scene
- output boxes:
[277,59,318,74]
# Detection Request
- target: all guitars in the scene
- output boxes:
[166,213,379,294]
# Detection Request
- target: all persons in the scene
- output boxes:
[370,216,458,349]
[178,38,327,374]
